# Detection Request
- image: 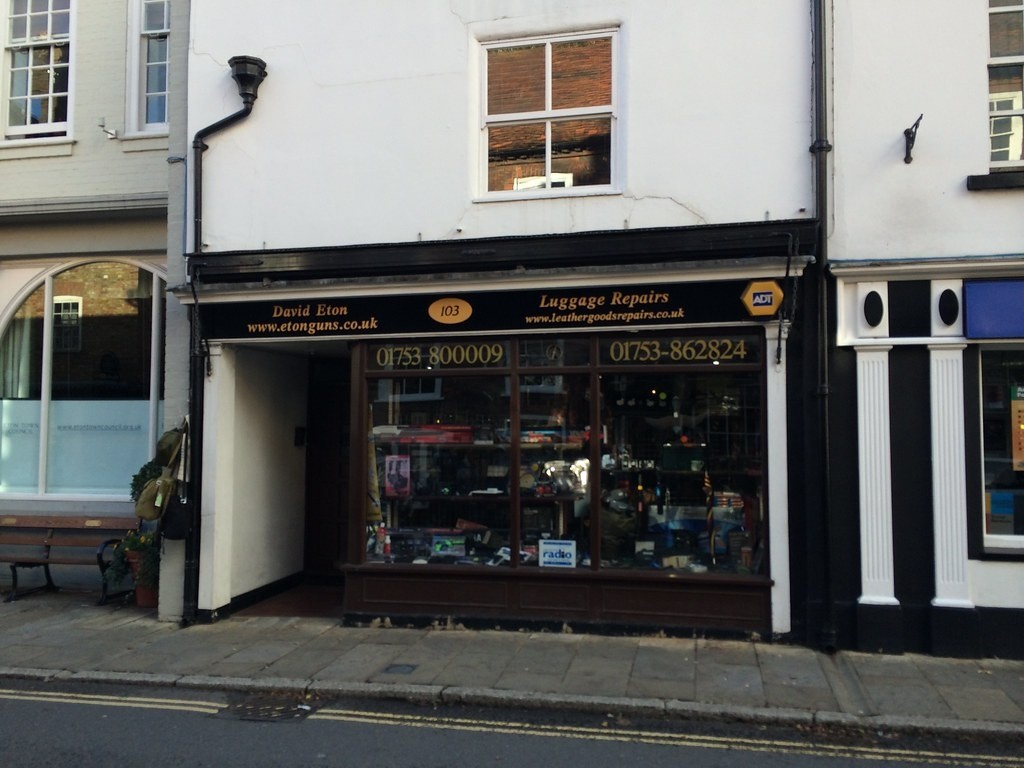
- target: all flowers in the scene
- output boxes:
[103,528,160,592]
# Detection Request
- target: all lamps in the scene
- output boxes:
[98,117,117,139]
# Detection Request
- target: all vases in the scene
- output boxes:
[126,550,157,608]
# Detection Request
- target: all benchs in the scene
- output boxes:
[0,514,144,606]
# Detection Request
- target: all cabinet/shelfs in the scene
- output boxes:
[339,342,778,638]
